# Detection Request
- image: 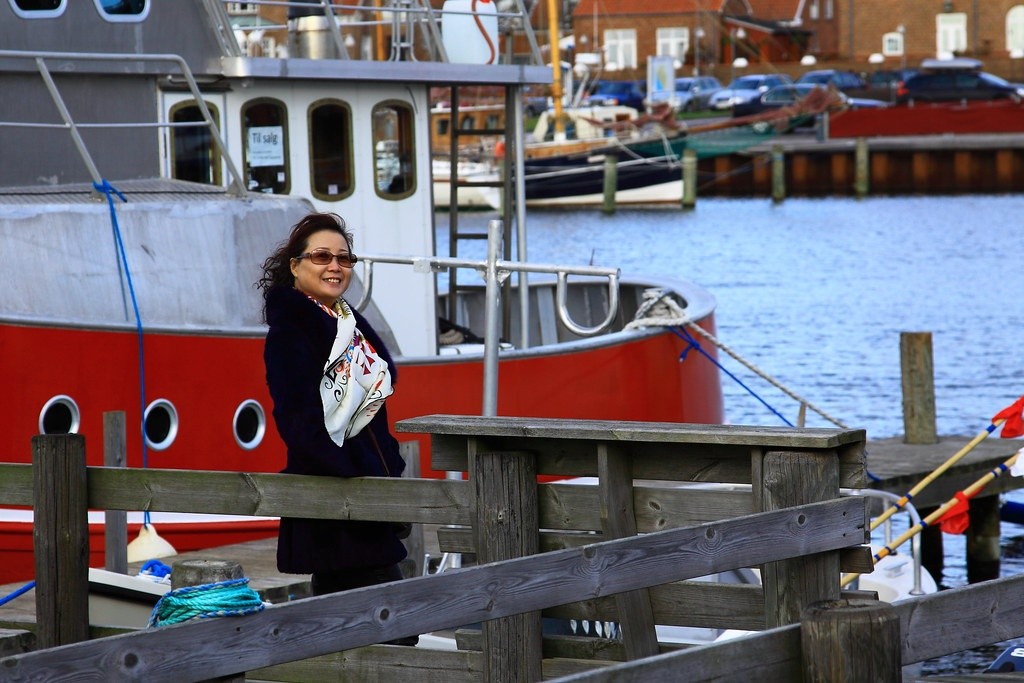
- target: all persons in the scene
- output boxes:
[257,212,419,646]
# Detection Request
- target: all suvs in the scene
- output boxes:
[892,56,1024,104]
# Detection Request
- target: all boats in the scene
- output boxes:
[0,0,726,600]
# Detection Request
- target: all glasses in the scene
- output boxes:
[300,249,358,269]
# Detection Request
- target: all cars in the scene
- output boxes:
[588,70,859,115]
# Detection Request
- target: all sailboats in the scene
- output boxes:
[470,0,850,213]
